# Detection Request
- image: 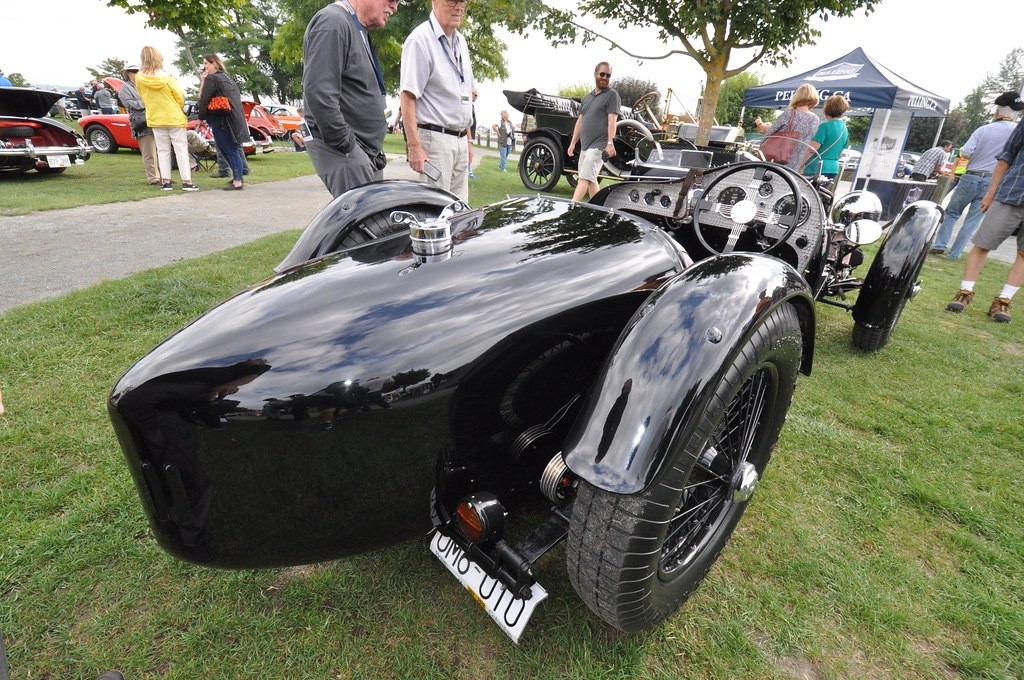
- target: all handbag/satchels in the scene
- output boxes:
[757,110,800,165]
[799,165,806,174]
[207,74,232,114]
[129,109,148,132]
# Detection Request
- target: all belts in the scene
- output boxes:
[966,170,992,177]
[417,124,467,137]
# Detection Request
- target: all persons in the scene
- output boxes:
[116,358,272,562]
[595,378,633,464]
[393,106,409,163]
[947,117,1024,323]
[292,107,309,153]
[347,209,487,264]
[118,46,251,191]
[521,114,537,172]
[45,81,113,122]
[400,0,474,204]
[492,110,514,173]
[753,270,803,318]
[568,62,621,202]
[928,91,1024,261]
[468,89,479,178]
[946,146,970,195]
[261,372,442,429]
[756,83,849,189]
[300,0,413,198]
[911,140,952,182]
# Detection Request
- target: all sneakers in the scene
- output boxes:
[947,289,976,312]
[160,183,173,191]
[182,182,199,191]
[987,297,1012,323]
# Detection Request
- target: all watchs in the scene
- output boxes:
[468,139,474,145]
[608,141,614,145]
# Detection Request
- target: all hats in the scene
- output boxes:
[995,91,1024,111]
[121,64,140,82]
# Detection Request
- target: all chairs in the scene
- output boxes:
[187,131,220,171]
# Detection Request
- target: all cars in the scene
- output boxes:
[838,149,861,168]
[78,76,273,154]
[502,81,746,195]
[107,133,947,647]
[0,85,94,178]
[46,88,100,118]
[896,152,921,177]
[183,100,308,142]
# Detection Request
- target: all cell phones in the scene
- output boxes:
[423,160,442,181]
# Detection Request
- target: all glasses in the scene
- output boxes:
[447,0,468,7]
[596,72,611,78]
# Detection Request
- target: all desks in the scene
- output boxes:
[855,178,937,220]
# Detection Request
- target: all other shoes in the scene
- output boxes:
[63,119,73,122]
[468,172,480,179]
[243,171,250,175]
[209,174,231,178]
[929,249,944,254]
[498,166,507,173]
[222,178,244,191]
[191,166,199,173]
[151,178,176,185]
[301,145,306,152]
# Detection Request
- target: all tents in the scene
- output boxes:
[737,46,951,190]
